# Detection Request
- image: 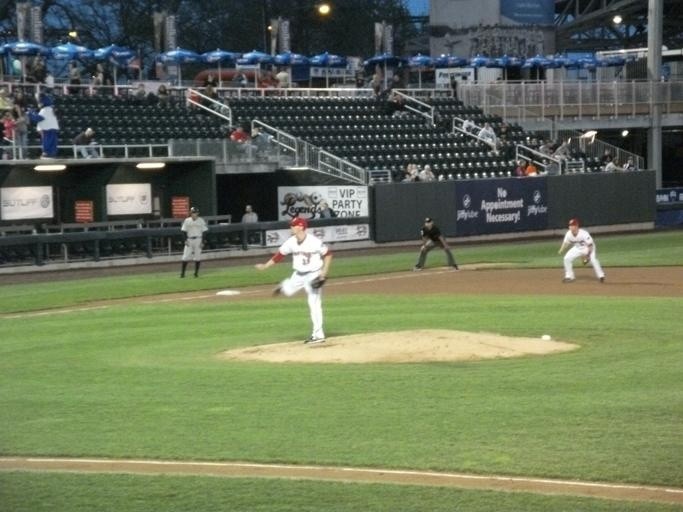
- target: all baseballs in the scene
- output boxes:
[542,334,550,339]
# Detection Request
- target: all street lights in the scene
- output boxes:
[260,4,330,57]
[592,11,623,50]
[38,4,77,84]
[617,129,629,149]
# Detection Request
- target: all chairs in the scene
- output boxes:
[17,95,609,183]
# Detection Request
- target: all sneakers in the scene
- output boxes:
[304,336,325,344]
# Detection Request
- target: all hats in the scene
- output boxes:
[569,218,579,226]
[289,217,306,230]
[191,207,199,213]
[425,217,431,221]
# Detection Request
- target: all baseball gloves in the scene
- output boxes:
[312,275,324,290]
[578,255,592,264]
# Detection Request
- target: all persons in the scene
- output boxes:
[557,218,606,284]
[242,205,257,223]
[180,206,209,278]
[404,163,435,180]
[230,126,246,144]
[462,116,638,177]
[0,57,221,159]
[354,64,410,118]
[254,217,333,344]
[410,218,458,272]
